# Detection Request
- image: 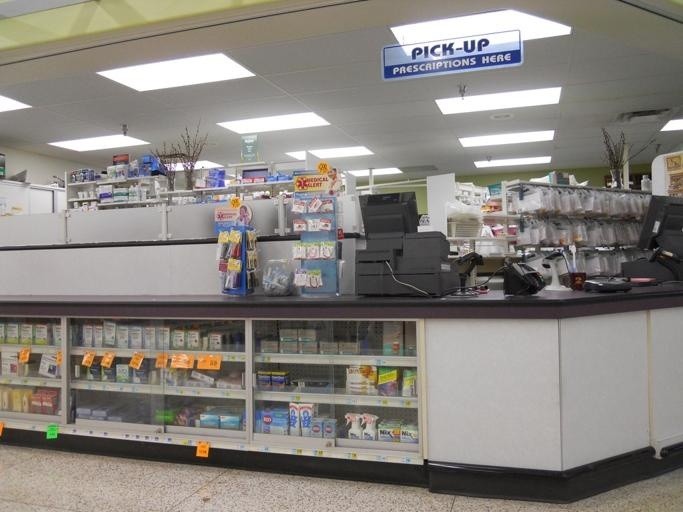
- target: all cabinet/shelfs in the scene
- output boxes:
[562,288,682,503]
[62,169,362,214]
[1,292,562,503]
[500,180,652,282]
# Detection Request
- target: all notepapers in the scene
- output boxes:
[197,354,221,371]
[81,351,96,368]
[101,351,116,368]
[46,423,58,439]
[170,352,195,369]
[57,351,64,364]
[128,351,145,371]
[196,441,210,458]
[155,352,168,370]
[18,347,30,363]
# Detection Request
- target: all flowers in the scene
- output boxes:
[149,140,178,179]
[596,122,660,168]
[171,117,210,176]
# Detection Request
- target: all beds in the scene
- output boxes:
[342,167,403,177]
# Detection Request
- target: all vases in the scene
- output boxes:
[610,168,625,190]
[184,175,194,190]
[168,177,176,192]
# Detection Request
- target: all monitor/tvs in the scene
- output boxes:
[358,191,419,240]
[636,195,683,251]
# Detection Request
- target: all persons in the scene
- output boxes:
[488,197,503,212]
[235,206,250,226]
